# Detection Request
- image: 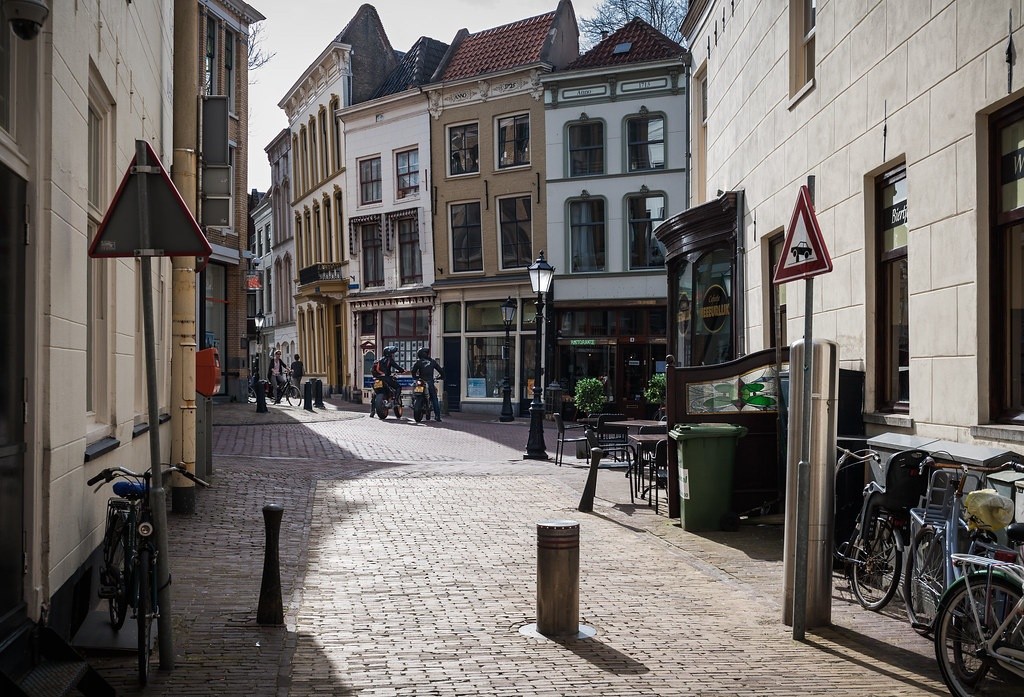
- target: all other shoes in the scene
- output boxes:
[435,415,442,422]
[273,389,277,396]
[370,411,375,418]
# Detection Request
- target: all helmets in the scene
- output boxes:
[417,347,429,359]
[383,346,398,357]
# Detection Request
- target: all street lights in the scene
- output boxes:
[251,308,266,397]
[499,296,519,422]
[521,250,557,461]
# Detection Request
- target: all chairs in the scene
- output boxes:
[860,449,933,546]
[552,412,668,511]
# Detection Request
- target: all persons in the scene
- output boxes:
[370,346,407,418]
[251,366,259,398]
[411,348,445,422]
[267,350,293,403]
[290,354,303,398]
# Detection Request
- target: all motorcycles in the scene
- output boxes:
[371,370,407,419]
[409,366,446,423]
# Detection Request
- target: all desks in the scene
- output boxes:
[628,434,667,497]
[578,417,634,465]
[602,420,667,477]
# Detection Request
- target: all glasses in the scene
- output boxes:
[277,354,281,355]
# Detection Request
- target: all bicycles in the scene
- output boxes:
[85,461,211,688]
[265,369,302,406]
[904,456,1024,687]
[932,521,1024,697]
[247,381,257,403]
[833,444,931,612]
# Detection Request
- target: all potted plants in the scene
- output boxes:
[573,375,609,458]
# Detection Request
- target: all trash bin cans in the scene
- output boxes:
[670,421,747,531]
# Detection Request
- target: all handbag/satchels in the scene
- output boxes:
[302,367,305,375]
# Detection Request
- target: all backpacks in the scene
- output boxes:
[371,357,389,378]
